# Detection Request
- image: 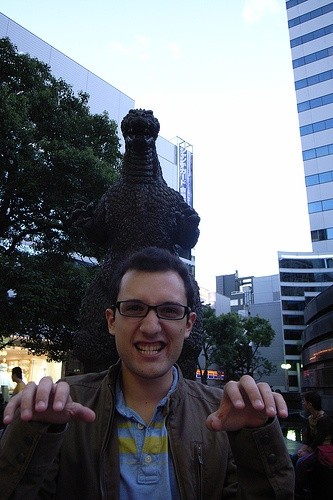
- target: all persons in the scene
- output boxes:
[10,367,25,399]
[290,392,333,500]
[0,247,295,500]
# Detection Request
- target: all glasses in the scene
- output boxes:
[110,299,191,326]
